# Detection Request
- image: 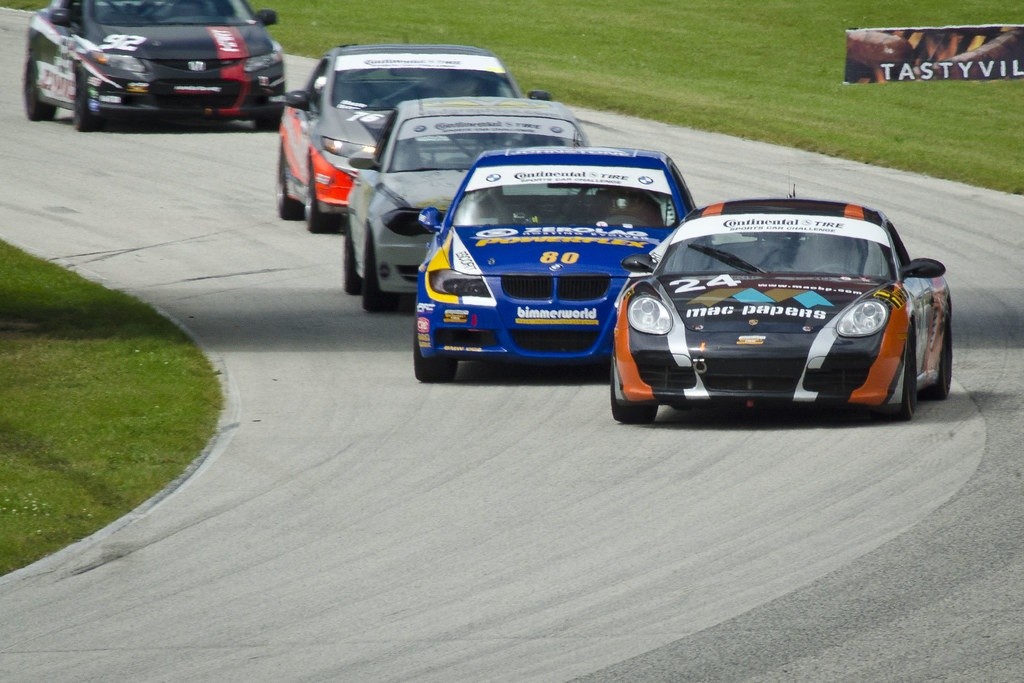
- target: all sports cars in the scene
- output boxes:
[22,1,286,126]
[609,197,955,426]
[272,42,555,235]
[343,93,594,311]
[412,147,703,384]
